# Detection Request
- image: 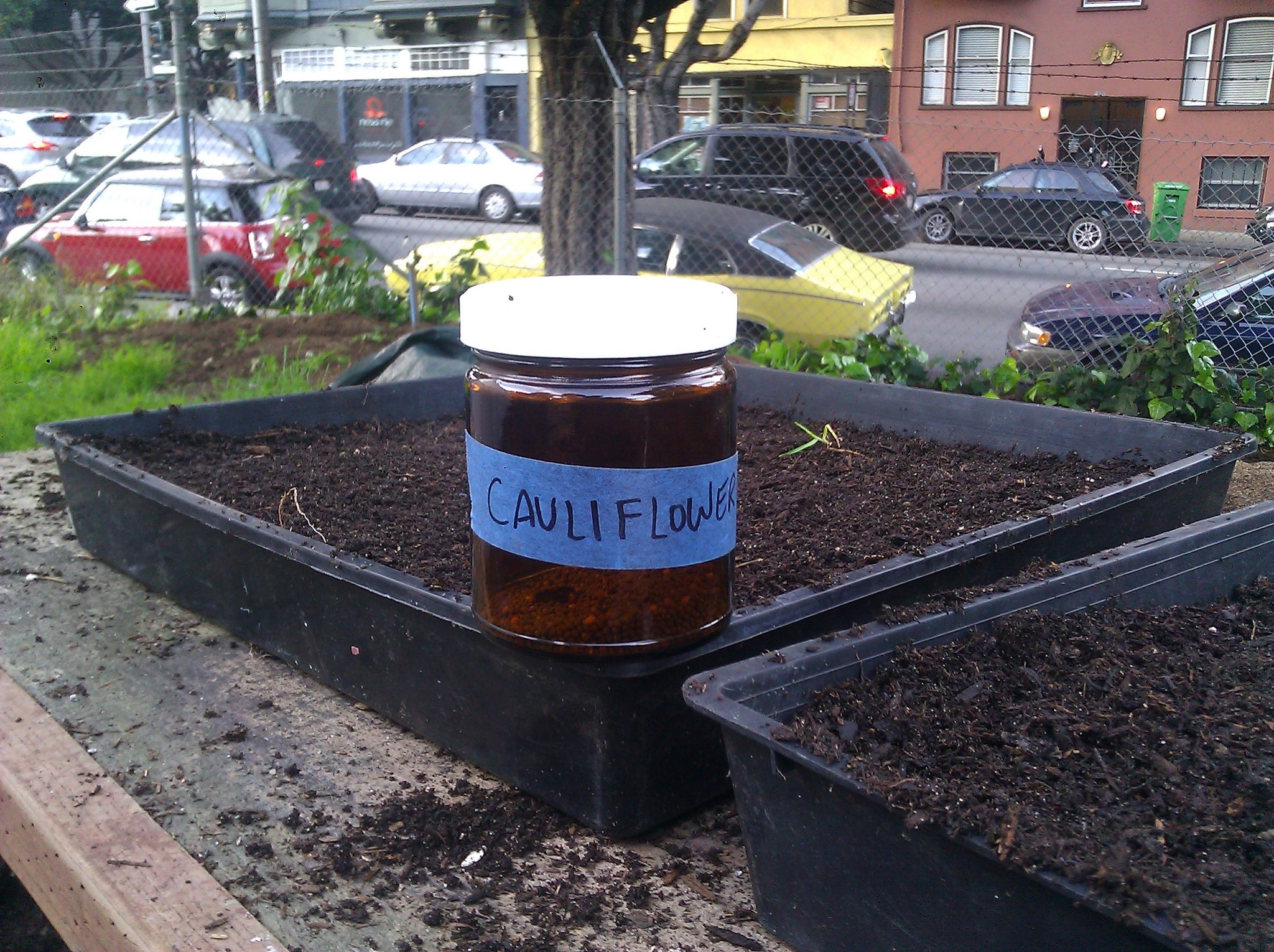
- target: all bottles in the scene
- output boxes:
[459,275,742,656]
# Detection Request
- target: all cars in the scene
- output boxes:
[384,197,916,356]
[1007,242,1274,378]
[1245,202,1274,245]
[15,115,363,226]
[0,164,35,250]
[359,138,545,225]
[0,109,91,186]
[0,167,351,318]
[916,163,1151,254]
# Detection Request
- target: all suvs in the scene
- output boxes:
[631,123,918,251]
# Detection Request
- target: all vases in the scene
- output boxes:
[683,497,1272,952]
[25,366,1257,835]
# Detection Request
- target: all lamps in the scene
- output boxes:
[1155,107,1166,121]
[1039,106,1048,120]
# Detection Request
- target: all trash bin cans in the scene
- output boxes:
[1149,181,1191,242]
